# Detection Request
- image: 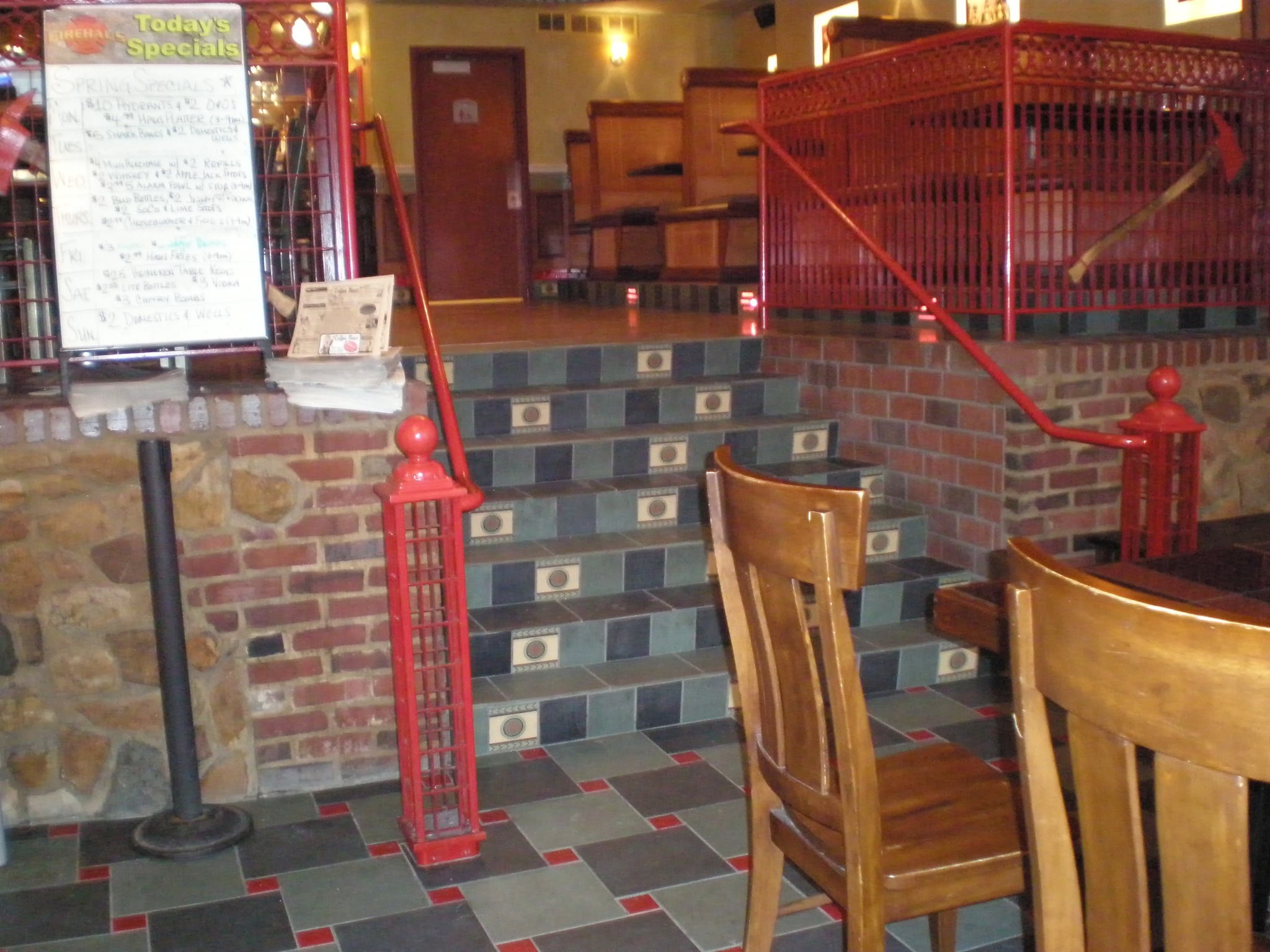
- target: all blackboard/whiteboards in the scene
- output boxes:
[40,0,273,353]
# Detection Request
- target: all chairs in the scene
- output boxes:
[0,103,317,385]
[704,445,1030,952]
[1009,537,1270,952]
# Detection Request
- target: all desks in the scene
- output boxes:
[736,141,824,157]
[935,540,1270,658]
[626,162,682,177]
[909,103,1241,162]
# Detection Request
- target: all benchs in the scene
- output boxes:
[536,18,1270,282]
